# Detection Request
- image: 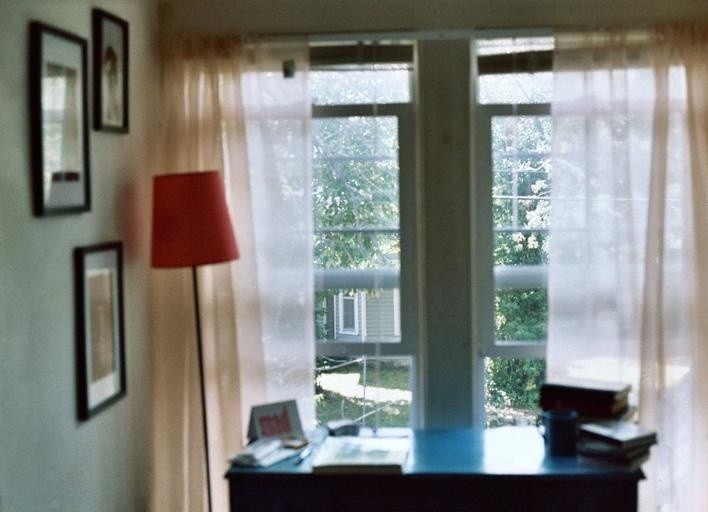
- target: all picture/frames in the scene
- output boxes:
[71,235,129,425]
[89,4,132,137]
[23,18,93,220]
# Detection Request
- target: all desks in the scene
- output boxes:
[219,423,647,512]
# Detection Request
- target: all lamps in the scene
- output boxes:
[146,166,241,512]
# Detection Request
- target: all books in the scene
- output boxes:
[538,377,659,467]
[313,435,412,476]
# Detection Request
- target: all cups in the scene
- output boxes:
[534,408,578,459]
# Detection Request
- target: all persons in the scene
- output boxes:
[103,44,122,124]
